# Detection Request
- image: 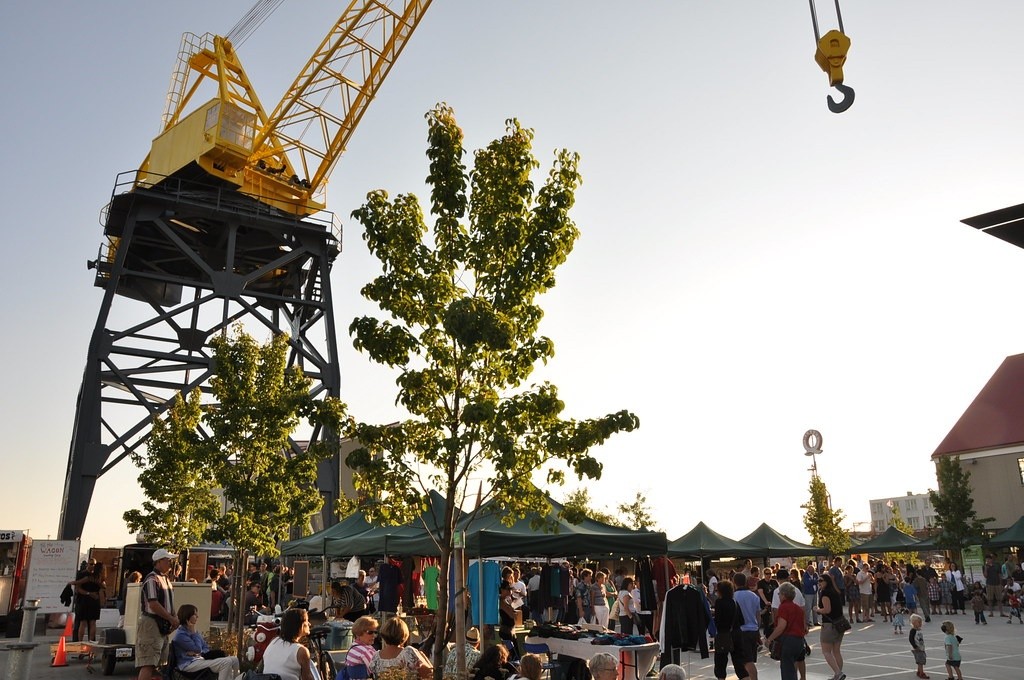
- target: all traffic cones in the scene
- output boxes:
[60,613,73,638]
[50,640,69,668]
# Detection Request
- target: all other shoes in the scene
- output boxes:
[832,671,846,680]
[1019,619,1024,623]
[999,614,1009,617]
[847,610,905,635]
[925,611,994,625]
[814,622,821,626]
[1006,620,1011,623]
[917,672,930,679]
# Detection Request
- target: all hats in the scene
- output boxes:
[153,549,179,561]
[464,627,481,643]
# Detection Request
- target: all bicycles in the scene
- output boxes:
[271,607,337,679]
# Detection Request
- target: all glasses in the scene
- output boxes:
[763,572,772,576]
[89,563,96,565]
[816,579,825,583]
[599,665,618,672]
[364,630,380,635]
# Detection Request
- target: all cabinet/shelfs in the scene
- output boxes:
[126,582,212,645]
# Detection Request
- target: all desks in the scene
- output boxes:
[494,625,539,661]
[524,635,661,680]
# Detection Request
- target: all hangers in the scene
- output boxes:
[477,556,489,564]
[386,552,409,567]
[640,551,693,587]
[543,558,566,569]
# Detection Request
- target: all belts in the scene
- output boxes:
[143,612,158,618]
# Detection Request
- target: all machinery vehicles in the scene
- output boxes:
[1,0,858,642]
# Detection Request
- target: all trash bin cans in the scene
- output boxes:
[321,622,353,650]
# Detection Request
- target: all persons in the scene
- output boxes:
[118,571,143,627]
[134,549,1024,680]
[73,558,107,642]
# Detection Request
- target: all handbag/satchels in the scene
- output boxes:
[832,616,851,632]
[201,648,228,659]
[631,612,640,623]
[156,617,173,635]
[609,591,622,620]
[770,640,783,660]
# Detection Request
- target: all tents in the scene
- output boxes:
[981,516,1024,566]
[281,480,935,656]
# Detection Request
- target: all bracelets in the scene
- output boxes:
[86,592,89,595]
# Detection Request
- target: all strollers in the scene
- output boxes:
[892,584,910,615]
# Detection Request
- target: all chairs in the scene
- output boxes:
[165,641,218,680]
[503,639,522,662]
[521,642,562,680]
